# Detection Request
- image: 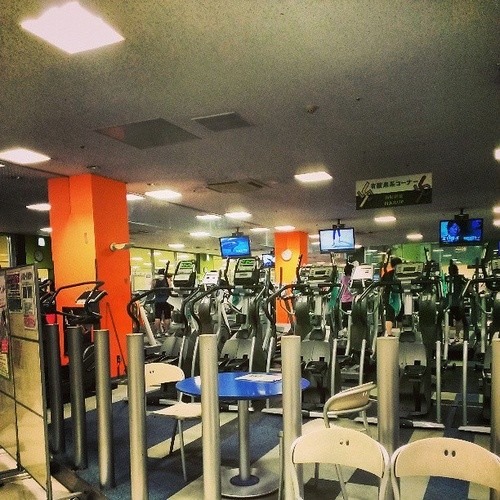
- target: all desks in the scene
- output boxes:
[175,371,314,499]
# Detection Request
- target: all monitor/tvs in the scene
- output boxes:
[319,228,355,253]
[440,218,484,247]
[219,235,252,259]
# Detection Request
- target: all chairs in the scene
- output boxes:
[143,361,202,483]
[392,436,500,500]
[288,426,391,500]
[300,382,377,500]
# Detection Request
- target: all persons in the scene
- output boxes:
[442,220,481,242]
[332,229,341,246]
[341,264,353,338]
[382,256,402,337]
[151,268,174,337]
[447,263,473,338]
